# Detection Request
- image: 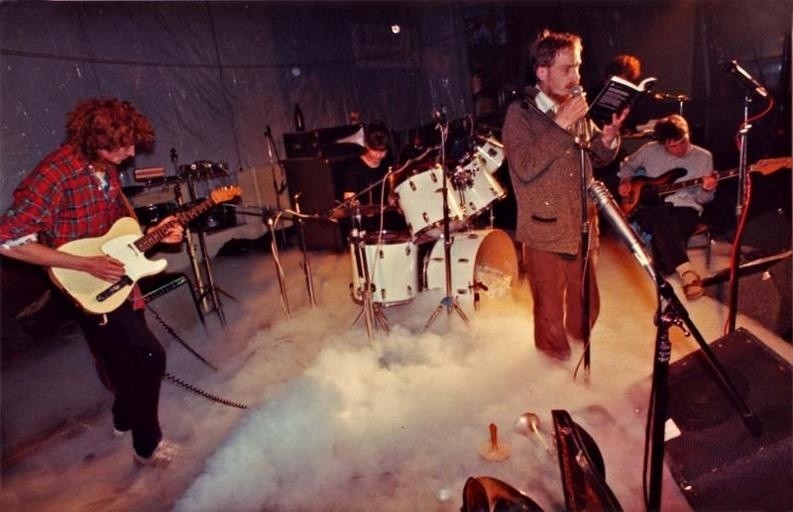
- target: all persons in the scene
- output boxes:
[334,127,402,234]
[581,55,644,104]
[500,31,631,366]
[1,94,184,464]
[616,111,719,301]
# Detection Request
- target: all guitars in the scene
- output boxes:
[619,157,793,217]
[47,185,243,314]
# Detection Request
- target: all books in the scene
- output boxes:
[584,73,659,125]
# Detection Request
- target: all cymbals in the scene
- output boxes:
[328,204,396,218]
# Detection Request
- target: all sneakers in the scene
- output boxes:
[112,420,201,474]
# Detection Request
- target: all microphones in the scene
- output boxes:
[587,181,656,280]
[170,147,180,173]
[727,60,768,97]
[571,86,587,149]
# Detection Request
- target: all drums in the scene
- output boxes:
[180,198,236,232]
[134,203,177,226]
[422,230,520,312]
[447,156,508,220]
[350,230,419,308]
[472,134,506,173]
[392,168,465,245]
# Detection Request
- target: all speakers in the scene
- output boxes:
[699,250,791,336]
[628,327,792,512]
[716,207,793,258]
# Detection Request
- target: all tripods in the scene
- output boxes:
[186,175,237,330]
[426,128,468,328]
[349,202,389,337]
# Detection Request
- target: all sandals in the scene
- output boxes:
[681,270,704,300]
